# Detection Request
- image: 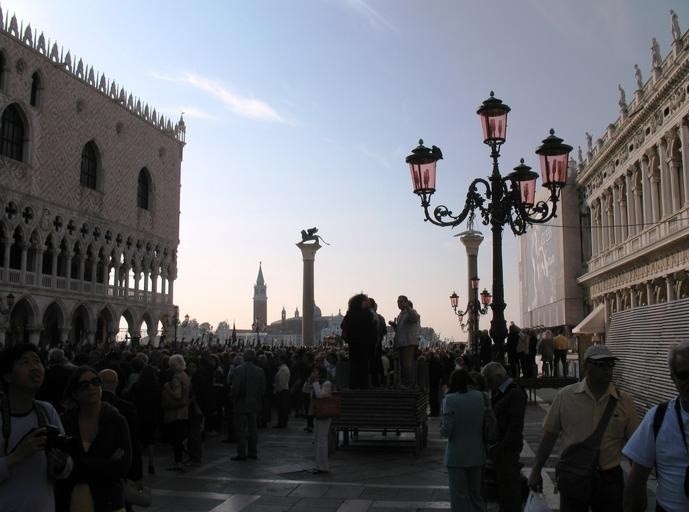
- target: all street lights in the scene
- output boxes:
[251,316,268,345]
[163,304,190,354]
[405,87,574,377]
[449,276,493,360]
[0,292,16,322]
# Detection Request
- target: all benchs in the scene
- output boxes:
[328,390,427,455]
[521,376,578,402]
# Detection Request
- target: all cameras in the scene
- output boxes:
[37,423,60,451]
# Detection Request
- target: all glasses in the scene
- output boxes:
[76,376,103,392]
[586,360,616,368]
[672,367,689,381]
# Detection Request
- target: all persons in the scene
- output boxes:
[442,321,569,511]
[529,344,641,511]
[1,334,349,510]
[339,294,466,419]
[622,340,688,511]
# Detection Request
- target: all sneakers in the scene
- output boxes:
[427,411,442,417]
[165,459,202,471]
[304,427,315,433]
[303,468,329,476]
[230,454,258,463]
[270,423,289,431]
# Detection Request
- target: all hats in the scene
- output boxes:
[584,343,621,362]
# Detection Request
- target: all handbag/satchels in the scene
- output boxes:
[311,378,343,420]
[120,477,153,508]
[556,388,621,503]
[481,391,509,465]
